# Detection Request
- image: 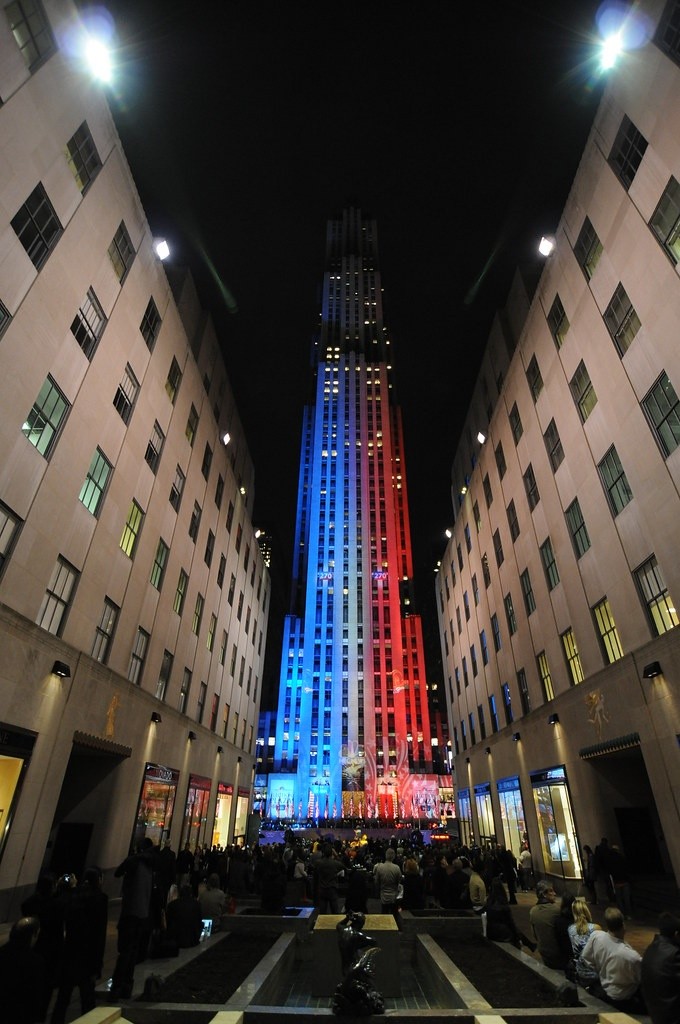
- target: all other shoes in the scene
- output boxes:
[509,901,517,904]
[529,943,537,952]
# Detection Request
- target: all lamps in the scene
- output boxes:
[152,237,171,261]
[548,713,559,725]
[189,731,196,740]
[220,430,230,446]
[477,430,488,444]
[539,233,556,258]
[51,660,71,677]
[643,662,663,678]
[512,732,520,741]
[151,712,162,723]
[218,746,223,753]
[445,528,454,539]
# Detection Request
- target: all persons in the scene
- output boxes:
[0,814,680,1024]
[332,910,385,1017]
